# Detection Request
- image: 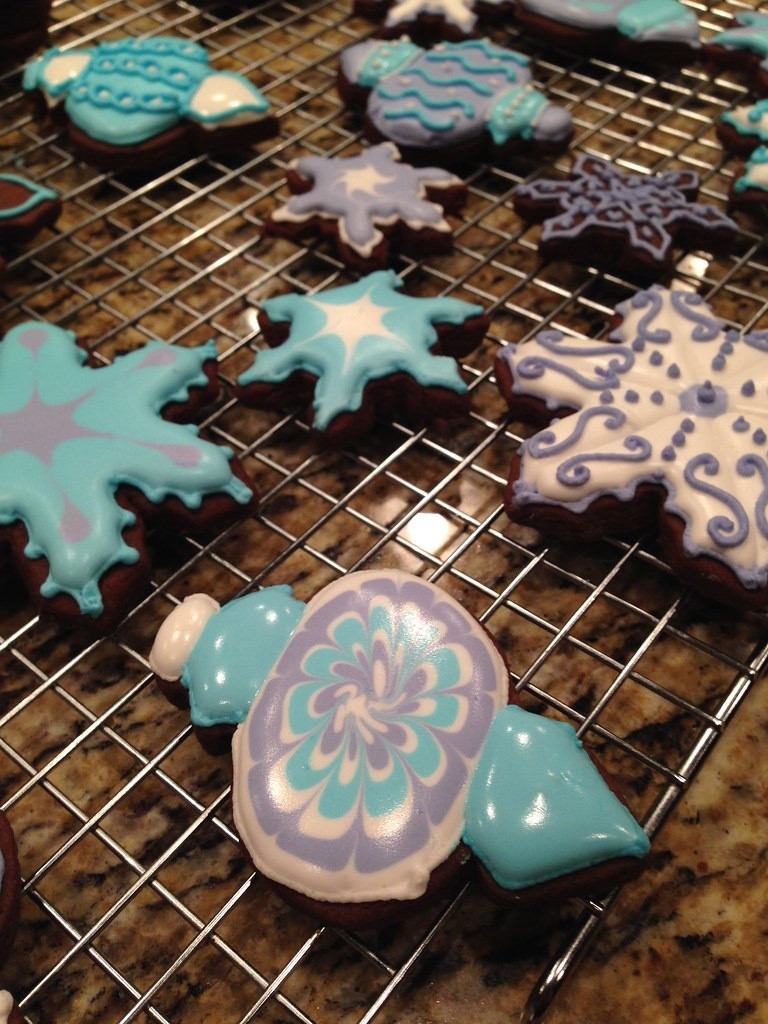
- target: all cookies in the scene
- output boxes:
[0,0,768,921]
